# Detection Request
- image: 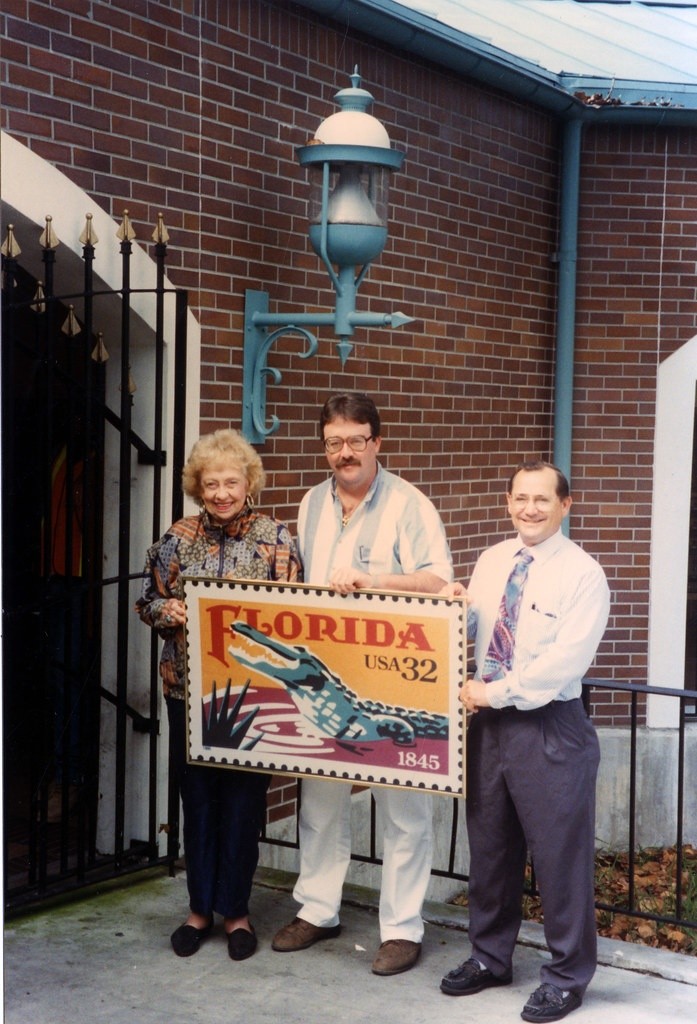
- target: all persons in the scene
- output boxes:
[436,460,610,1024]
[272,394,454,975]
[132,428,304,961]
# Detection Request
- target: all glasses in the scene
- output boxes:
[326,434,374,455]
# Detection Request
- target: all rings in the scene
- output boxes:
[164,615,171,623]
[462,697,468,703]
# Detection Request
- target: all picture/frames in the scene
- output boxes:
[180,576,468,801]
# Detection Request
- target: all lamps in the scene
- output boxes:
[242,65,415,445]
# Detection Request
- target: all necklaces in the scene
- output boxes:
[342,472,375,526]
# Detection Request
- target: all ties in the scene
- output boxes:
[481,549,534,682]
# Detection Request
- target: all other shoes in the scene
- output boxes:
[227,919,258,961]
[373,939,421,976]
[171,912,214,956]
[441,958,511,996]
[38,780,75,823]
[521,983,584,1023]
[272,919,341,952]
